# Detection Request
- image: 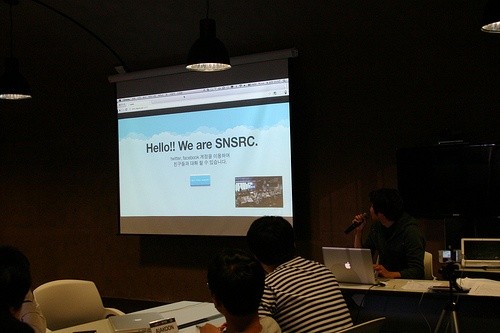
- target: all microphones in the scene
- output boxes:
[344,212,369,234]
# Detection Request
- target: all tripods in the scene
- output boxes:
[428,273,461,332]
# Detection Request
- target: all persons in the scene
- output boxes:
[352,187,426,280]
[246,216,354,333]
[200,248,282,333]
[0,244,36,333]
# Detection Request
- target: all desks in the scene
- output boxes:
[340,278,500,319]
[48,300,227,333]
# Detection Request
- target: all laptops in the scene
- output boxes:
[322,246,390,285]
[461,238,500,267]
[106,310,165,333]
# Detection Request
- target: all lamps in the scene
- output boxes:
[480,0,500,33]
[0,0,33,100]
[185,0,232,72]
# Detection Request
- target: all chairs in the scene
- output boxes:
[33,279,125,331]
